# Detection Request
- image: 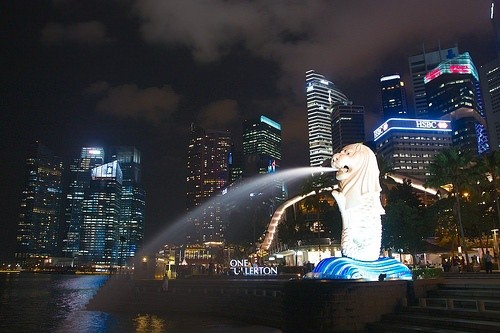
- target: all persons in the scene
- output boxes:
[442,255,478,273]
[162,273,169,293]
[203,260,287,279]
[484,251,493,274]
[109,266,113,278]
[302,261,312,276]
